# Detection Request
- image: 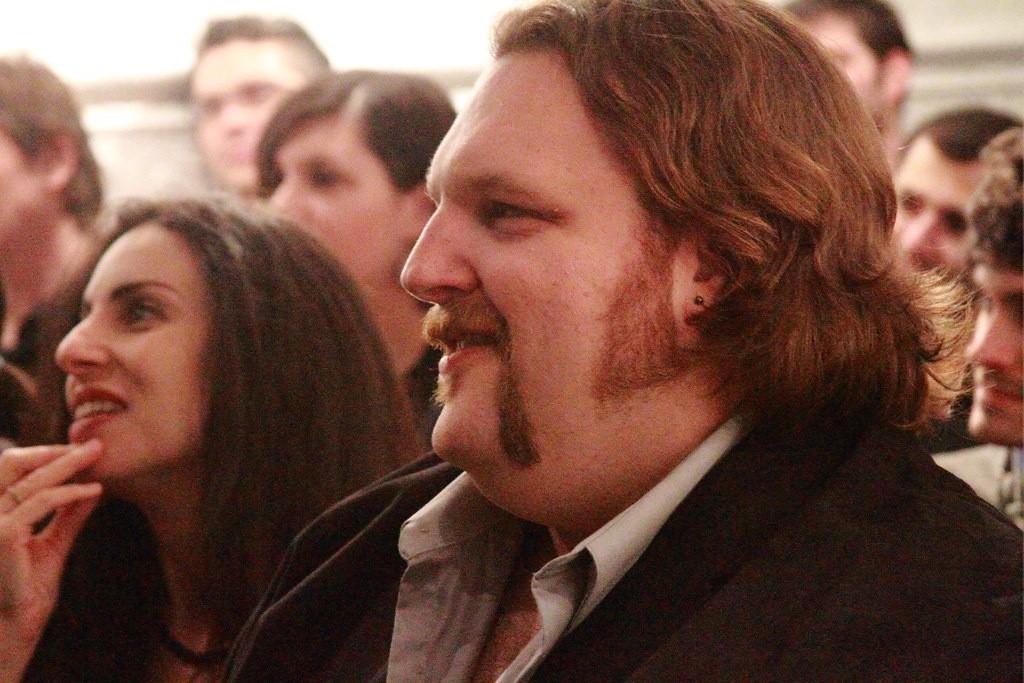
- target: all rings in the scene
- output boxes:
[7,487,22,505]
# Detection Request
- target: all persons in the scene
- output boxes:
[0,0,1024,537]
[0,194,430,683]
[215,0,1024,683]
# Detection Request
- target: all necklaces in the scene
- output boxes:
[158,618,228,667]
